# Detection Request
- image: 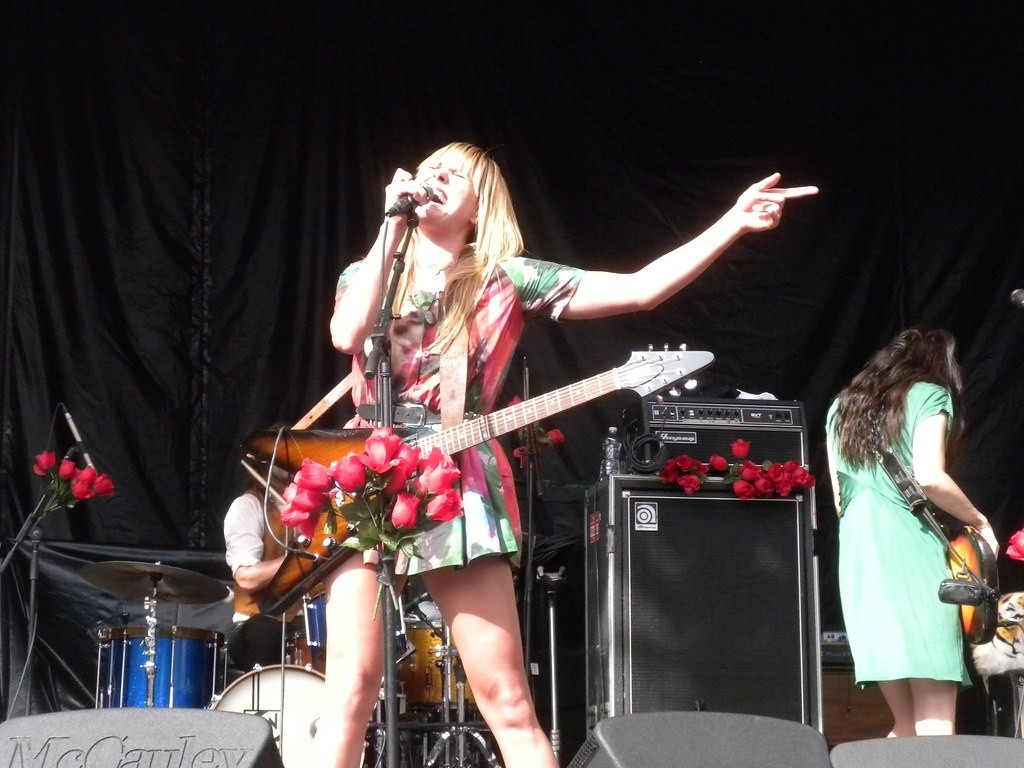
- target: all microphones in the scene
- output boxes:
[62,405,98,476]
[386,182,434,217]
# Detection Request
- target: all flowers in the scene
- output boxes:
[1006,526,1024,563]
[281,431,463,621]
[506,396,567,469]
[33,451,117,528]
[662,437,816,502]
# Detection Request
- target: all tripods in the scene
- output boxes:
[424,653,503,768]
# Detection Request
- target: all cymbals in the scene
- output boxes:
[78,560,230,602]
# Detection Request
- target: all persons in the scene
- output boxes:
[312,143,819,768]
[825,327,999,740]
[223,421,305,672]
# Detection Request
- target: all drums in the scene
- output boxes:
[394,618,480,711]
[306,589,406,674]
[96,625,227,708]
[209,663,369,768]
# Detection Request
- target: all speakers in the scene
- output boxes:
[829,734,1024,768]
[821,664,897,753]
[0,707,285,768]
[581,474,823,735]
[567,711,832,768]
[988,669,1024,739]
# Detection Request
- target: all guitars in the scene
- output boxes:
[243,340,715,620]
[945,524,999,645]
